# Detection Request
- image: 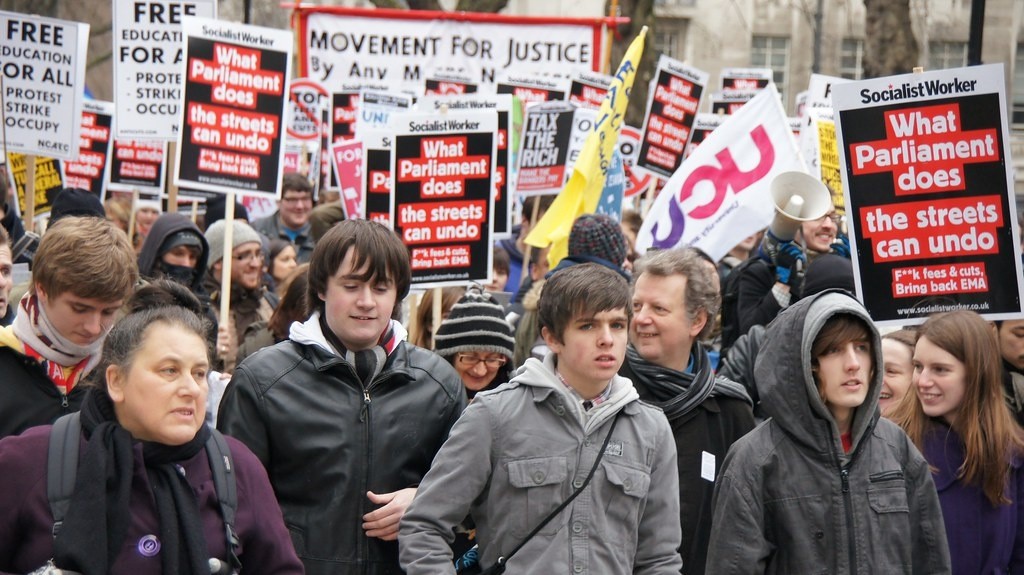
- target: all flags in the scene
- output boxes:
[523,32,643,269]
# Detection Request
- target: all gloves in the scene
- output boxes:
[775,242,806,284]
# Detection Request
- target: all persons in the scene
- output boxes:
[0,215,139,438]
[214,220,469,574]
[434,285,517,555]
[397,263,684,575]
[989,320,1024,428]
[704,289,952,574]
[0,279,306,575]
[0,173,1023,374]
[889,313,1024,575]
[876,331,918,418]
[617,251,756,575]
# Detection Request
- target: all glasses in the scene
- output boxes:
[816,212,844,226]
[281,196,311,206]
[457,350,508,371]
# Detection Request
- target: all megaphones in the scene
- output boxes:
[758,171,831,278]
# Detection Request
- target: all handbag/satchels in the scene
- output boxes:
[453,542,506,575]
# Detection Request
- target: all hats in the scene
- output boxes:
[202,221,262,272]
[569,214,626,271]
[48,187,105,221]
[159,232,204,256]
[434,283,515,366]
